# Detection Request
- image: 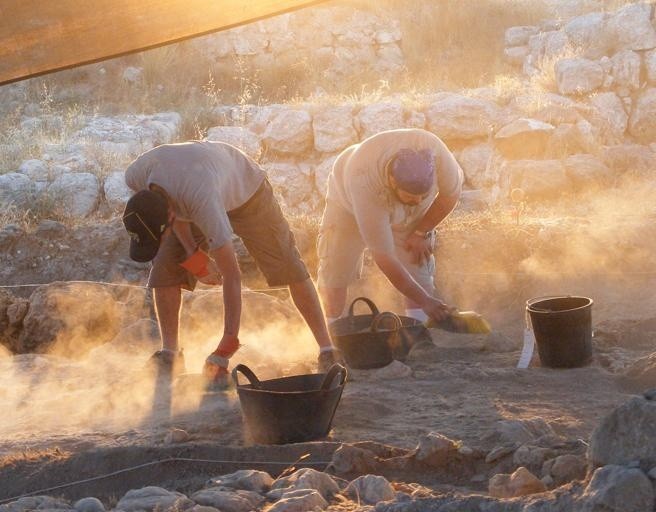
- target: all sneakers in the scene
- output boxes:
[142,347,186,378]
[317,350,353,382]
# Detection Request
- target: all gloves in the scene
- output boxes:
[201,336,244,390]
[178,248,224,286]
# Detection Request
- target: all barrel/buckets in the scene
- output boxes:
[527,296,594,368]
[330,295,424,370]
[233,362,348,443]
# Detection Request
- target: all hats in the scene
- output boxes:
[391,147,435,196]
[122,188,170,263]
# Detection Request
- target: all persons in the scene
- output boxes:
[122,140,353,383]
[315,129,464,326]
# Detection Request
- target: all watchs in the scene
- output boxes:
[415,230,434,239]
